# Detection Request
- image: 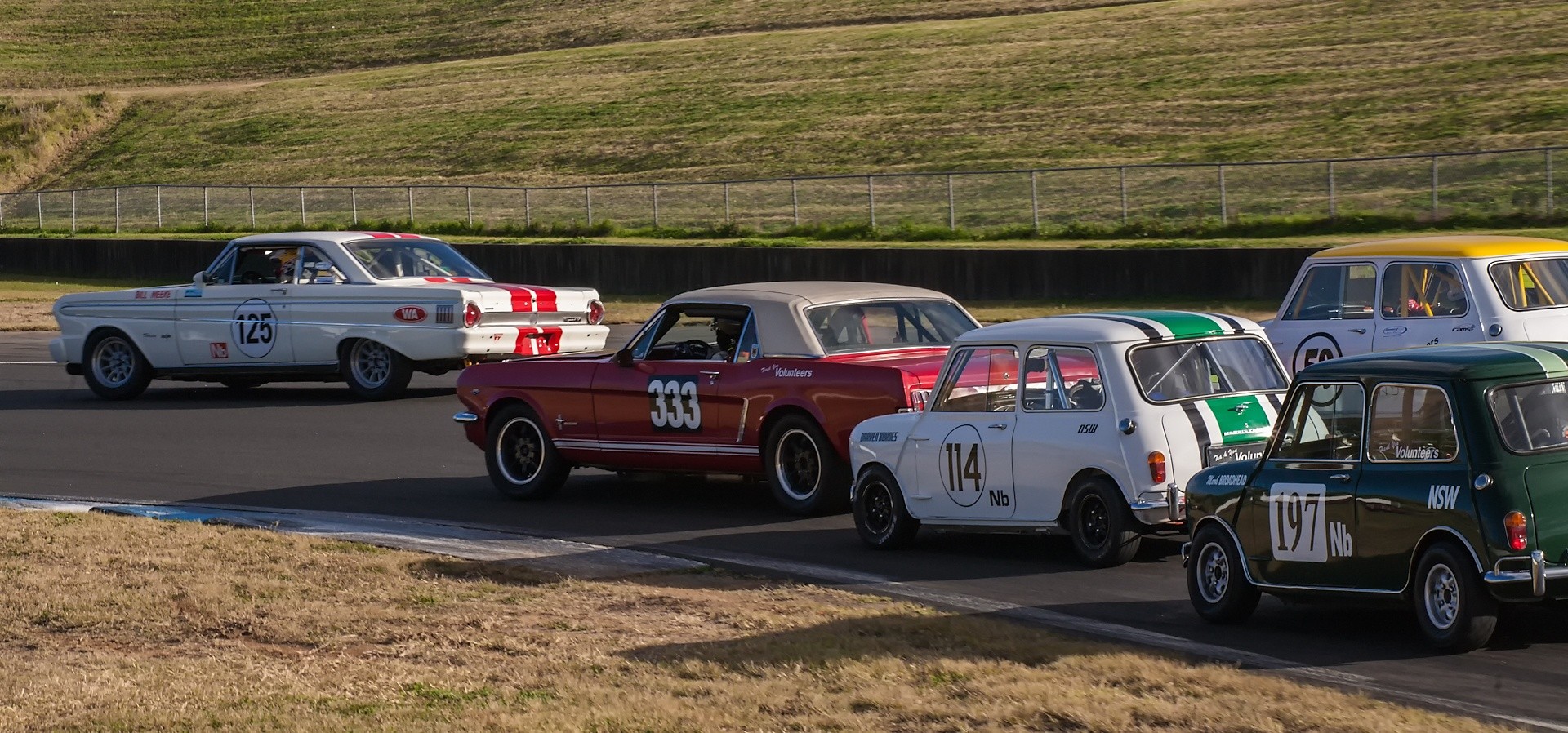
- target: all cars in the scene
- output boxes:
[1183,345,1568,652]
[849,310,1326,568]
[47,227,610,399]
[454,280,1099,517]
[1214,235,1568,426]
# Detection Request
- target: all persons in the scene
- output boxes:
[711,329,731,362]
